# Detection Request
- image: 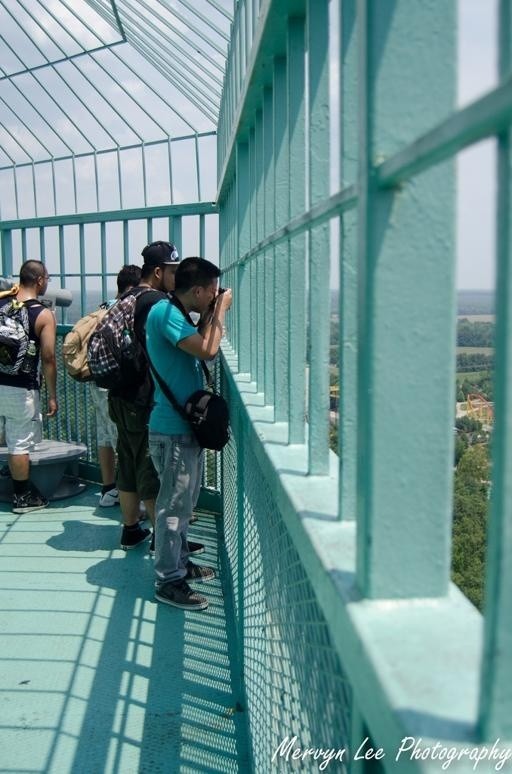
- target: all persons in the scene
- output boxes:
[108,240,181,551]
[0,260,60,513]
[88,264,143,508]
[0,283,19,299]
[145,256,233,612]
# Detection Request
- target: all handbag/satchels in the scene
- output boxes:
[185,389,229,451]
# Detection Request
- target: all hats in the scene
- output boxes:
[141,241,181,265]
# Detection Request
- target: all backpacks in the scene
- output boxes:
[0,299,46,374]
[62,287,159,390]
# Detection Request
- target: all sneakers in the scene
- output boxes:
[12,491,49,513]
[150,540,204,556]
[99,488,120,507]
[155,578,208,610]
[184,561,217,583]
[121,525,153,550]
